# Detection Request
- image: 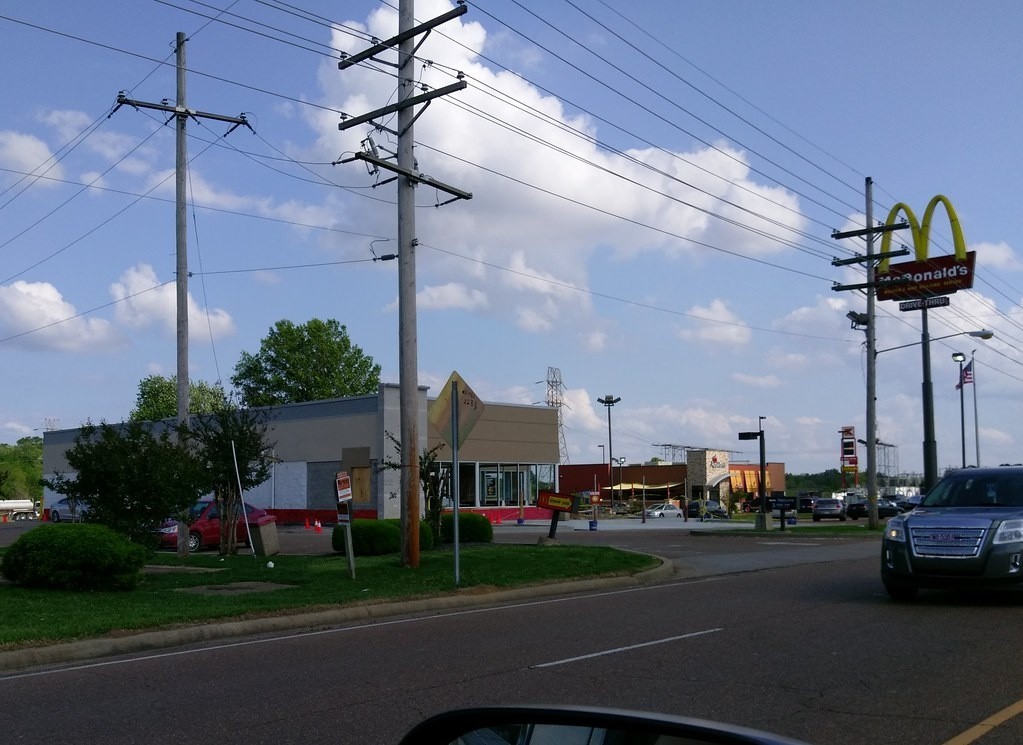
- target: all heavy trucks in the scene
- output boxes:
[0,498,40,519]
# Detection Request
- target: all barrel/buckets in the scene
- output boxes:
[589,521,597,531]
[788,517,796,524]
[517,519,524,524]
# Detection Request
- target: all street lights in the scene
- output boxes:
[612,456,626,502]
[865,328,996,530]
[597,445,605,465]
[596,396,623,503]
[758,415,767,433]
[950,351,967,468]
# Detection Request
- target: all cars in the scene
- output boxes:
[131,497,267,552]
[846,499,905,519]
[810,499,847,522]
[49,496,104,523]
[884,494,925,509]
[684,499,726,519]
[639,503,684,519]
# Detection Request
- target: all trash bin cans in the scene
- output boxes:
[251,514,280,558]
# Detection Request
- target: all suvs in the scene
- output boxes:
[880,463,1023,600]
